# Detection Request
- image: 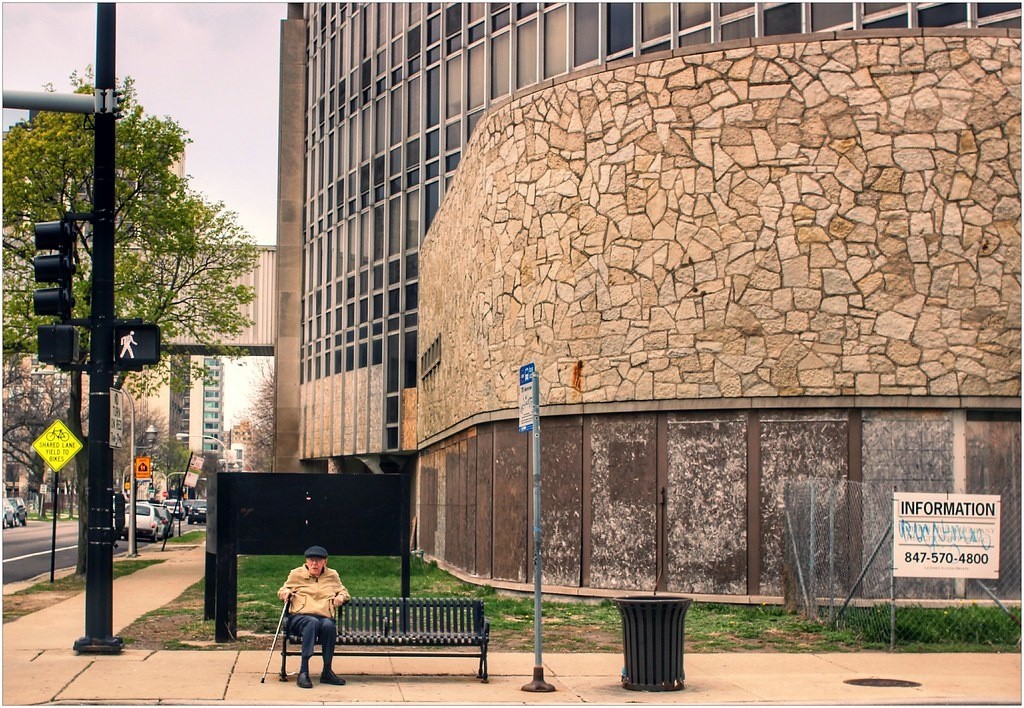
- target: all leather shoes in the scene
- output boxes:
[297,672,312,688]
[320,670,346,685]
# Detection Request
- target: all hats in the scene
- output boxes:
[304,546,328,558]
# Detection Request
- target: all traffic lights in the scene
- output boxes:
[31,219,78,318]
[115,322,163,366]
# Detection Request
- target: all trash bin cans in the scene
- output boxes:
[612,595,694,693]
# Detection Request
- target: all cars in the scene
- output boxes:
[187,500,207,525]
[182,499,196,517]
[2,496,16,530]
[163,500,185,518]
[112,496,176,542]
[6,497,28,527]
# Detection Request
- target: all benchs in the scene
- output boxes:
[280,596,491,684]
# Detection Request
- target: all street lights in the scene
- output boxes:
[30,369,139,556]
[174,433,229,474]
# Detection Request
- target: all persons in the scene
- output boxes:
[278,547,350,688]
